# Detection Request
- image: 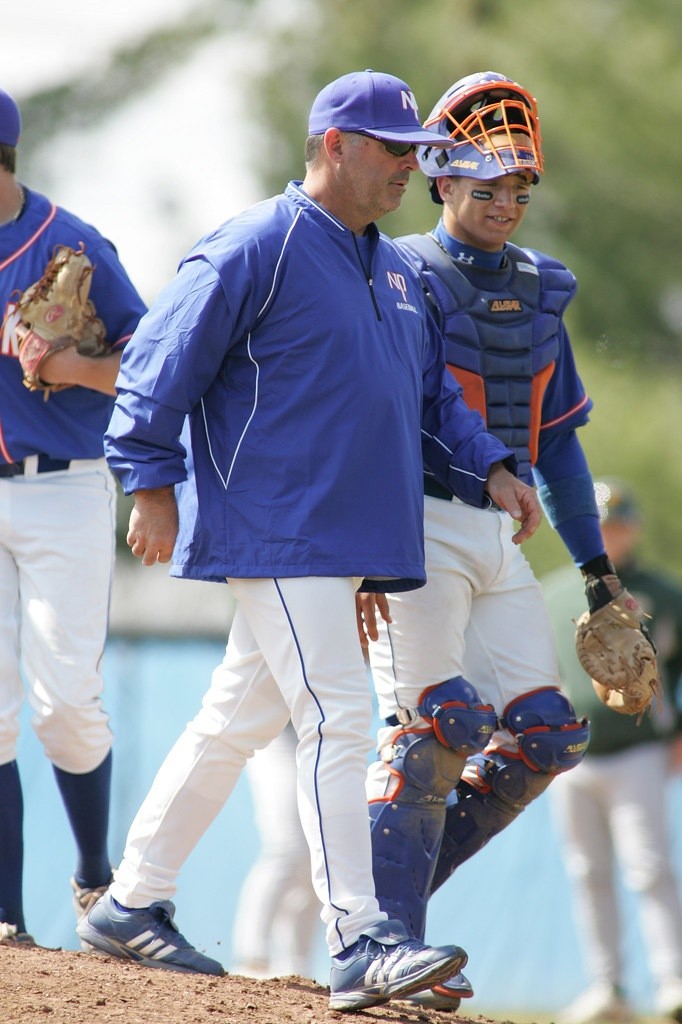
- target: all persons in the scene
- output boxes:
[235,720,321,985]
[355,71,661,1009]
[540,483,681,1023]
[76,66,542,1011]
[0,90,149,946]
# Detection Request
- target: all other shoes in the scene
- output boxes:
[656,977,682,1022]
[565,981,620,1024]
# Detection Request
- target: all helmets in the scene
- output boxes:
[416,71,545,185]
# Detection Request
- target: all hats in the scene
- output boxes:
[0,88,21,145]
[309,69,456,148]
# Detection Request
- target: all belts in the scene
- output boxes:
[421,471,503,511]
[0,452,70,478]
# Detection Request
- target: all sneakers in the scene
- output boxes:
[71,868,114,955]
[327,919,468,1012]
[75,883,226,979]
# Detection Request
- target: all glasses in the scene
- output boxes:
[347,129,420,158]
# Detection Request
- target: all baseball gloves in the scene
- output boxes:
[16,245,108,393]
[573,589,663,716]
